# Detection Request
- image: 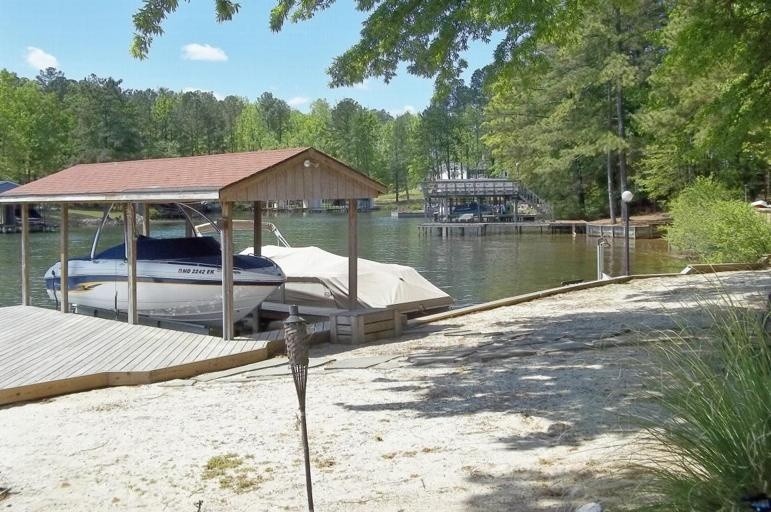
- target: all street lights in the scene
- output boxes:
[620,190,636,278]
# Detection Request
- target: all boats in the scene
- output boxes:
[45,201,289,328]
